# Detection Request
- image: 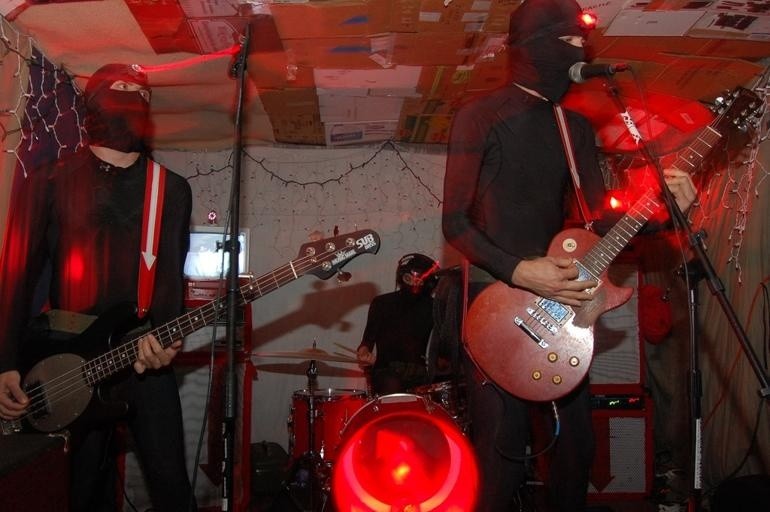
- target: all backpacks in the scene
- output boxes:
[331,394,484,512]
[407,380,472,426]
[288,389,368,483]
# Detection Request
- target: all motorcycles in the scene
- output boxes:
[273,385,331,511]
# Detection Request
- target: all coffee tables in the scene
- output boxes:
[251,353,361,363]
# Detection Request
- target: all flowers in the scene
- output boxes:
[568,62,629,85]
[226,30,249,80]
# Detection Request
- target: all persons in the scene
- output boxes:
[0,64,199,512]
[442,0,699,512]
[356,253,442,396]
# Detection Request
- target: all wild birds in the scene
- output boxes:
[182,225,253,280]
